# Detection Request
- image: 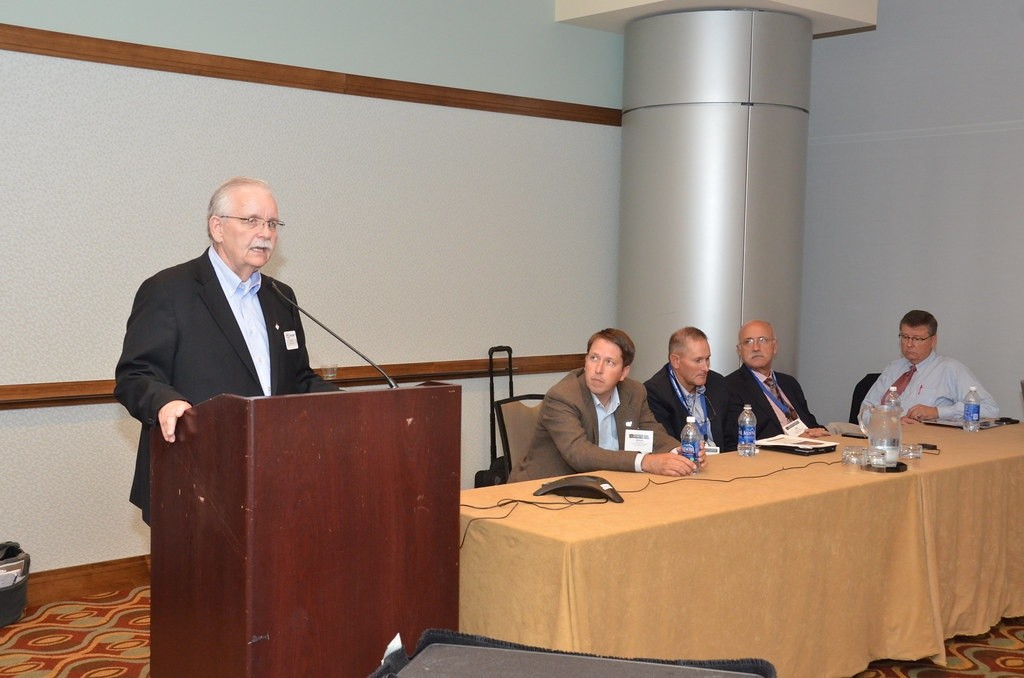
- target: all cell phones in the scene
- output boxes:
[995,419,1019,425]
[842,432,868,439]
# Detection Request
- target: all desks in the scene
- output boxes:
[460,422,1024,678]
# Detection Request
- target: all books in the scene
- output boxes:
[755,434,840,456]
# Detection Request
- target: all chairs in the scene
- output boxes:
[849,373,881,425]
[494,394,546,481]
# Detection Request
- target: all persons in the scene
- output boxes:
[507,328,708,484]
[641,326,741,454]
[113,177,348,529]
[724,319,832,441]
[826,310,999,439]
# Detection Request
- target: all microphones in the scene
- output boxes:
[266,276,398,388]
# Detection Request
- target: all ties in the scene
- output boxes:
[881,364,917,406]
[764,377,798,421]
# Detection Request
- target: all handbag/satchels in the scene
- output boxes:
[0,540,31,629]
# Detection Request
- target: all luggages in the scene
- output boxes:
[474,345,513,489]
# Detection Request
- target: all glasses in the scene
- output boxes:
[899,332,935,345]
[741,337,775,347]
[218,214,286,233]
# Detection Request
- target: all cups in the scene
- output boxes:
[841,444,922,476]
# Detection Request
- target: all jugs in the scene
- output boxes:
[858,400,904,450]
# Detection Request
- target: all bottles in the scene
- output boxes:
[737,405,756,456]
[885,387,901,406]
[962,387,981,431]
[681,416,701,472]
